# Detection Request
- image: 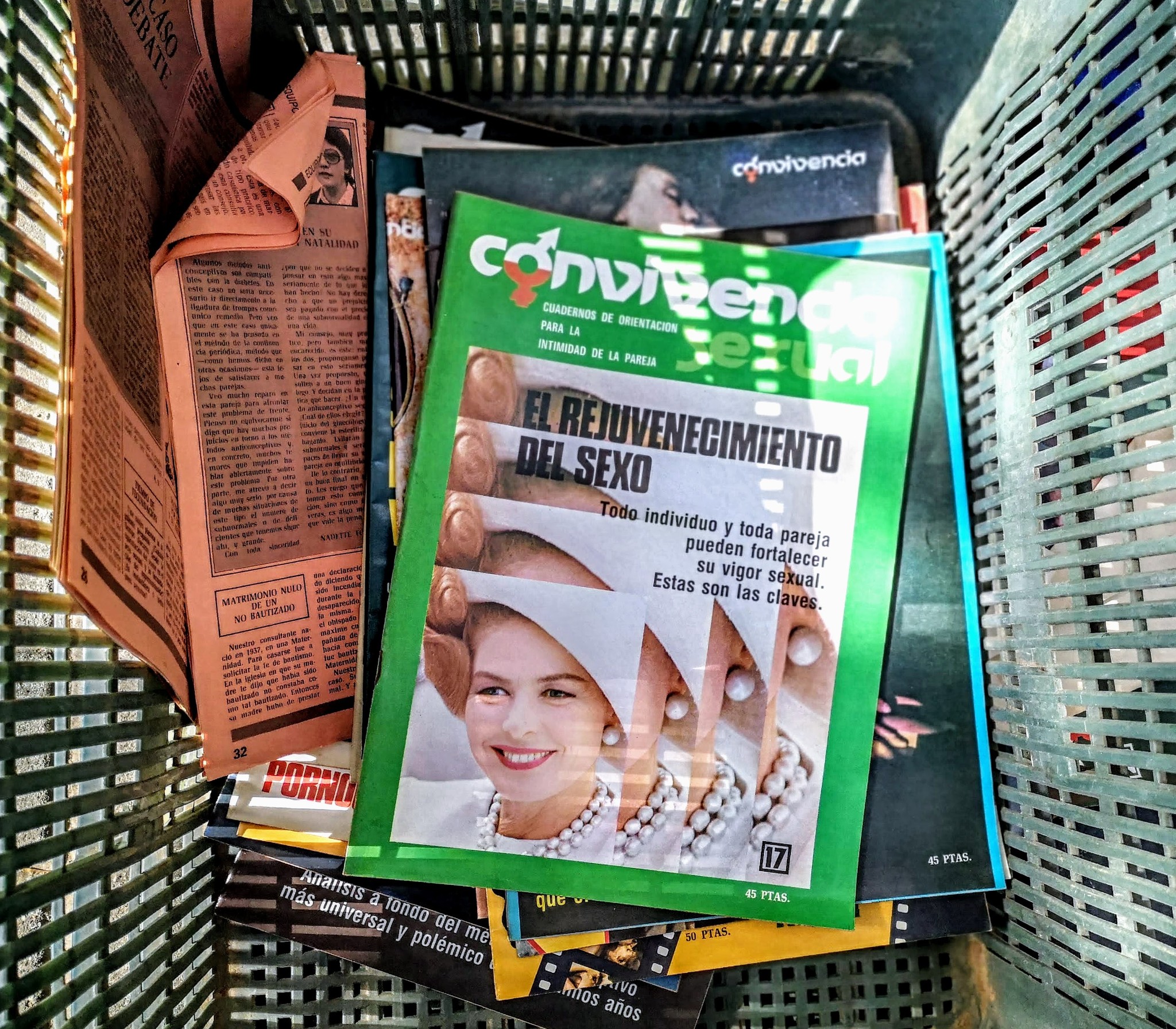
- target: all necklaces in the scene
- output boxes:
[749,767,808,858]
[682,763,736,843]
[681,787,742,875]
[752,734,802,818]
[477,790,614,859]
[483,779,608,857]
[615,769,674,846]
[614,788,680,867]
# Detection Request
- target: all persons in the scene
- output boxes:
[551,152,712,239]
[414,360,837,887]
[309,126,357,207]
[872,692,937,763]
[570,937,646,988]
